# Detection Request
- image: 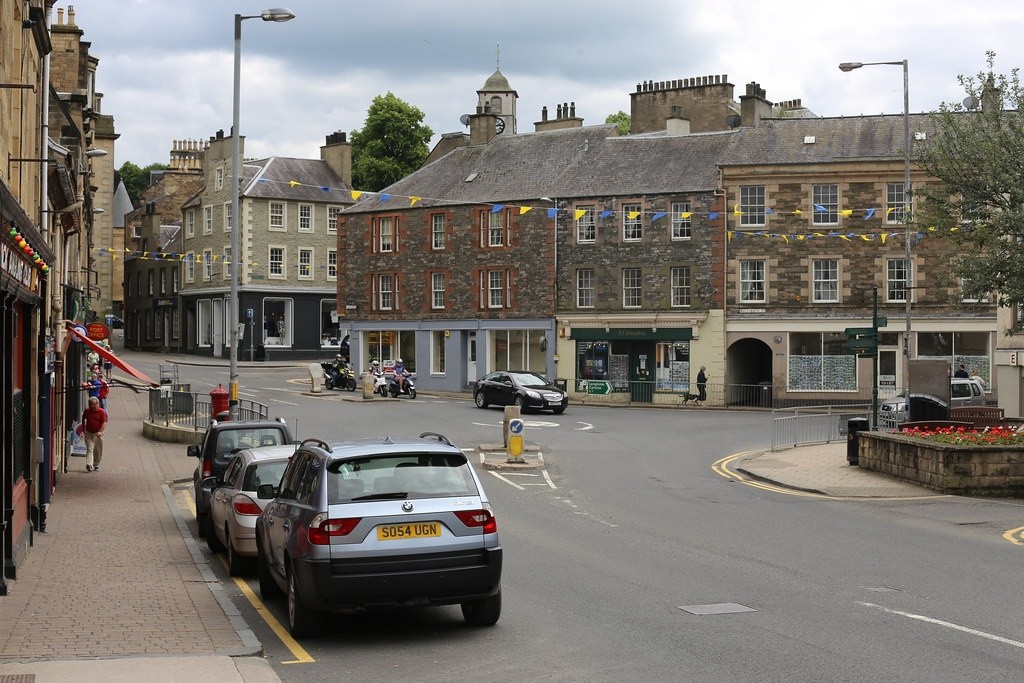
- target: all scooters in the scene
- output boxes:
[359,370,388,398]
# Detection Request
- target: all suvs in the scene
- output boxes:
[255,431,503,641]
[187,417,302,539]
[879,377,984,427]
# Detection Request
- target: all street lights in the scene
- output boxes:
[228,7,297,450]
[839,60,911,424]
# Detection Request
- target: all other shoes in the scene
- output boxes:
[336,384,340,388]
[94,466,99,470]
[400,389,404,393]
[86,464,92,472]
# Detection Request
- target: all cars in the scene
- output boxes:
[105,314,124,329]
[202,443,356,577]
[472,370,569,414]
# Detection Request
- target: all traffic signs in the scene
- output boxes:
[587,380,613,396]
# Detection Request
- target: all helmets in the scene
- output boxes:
[397,359,403,367]
[336,354,341,361]
[372,361,379,370]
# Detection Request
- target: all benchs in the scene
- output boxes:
[951,406,1006,428]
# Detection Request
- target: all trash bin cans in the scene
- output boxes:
[257,345,265,360]
[847,417,869,465]
[216,410,229,421]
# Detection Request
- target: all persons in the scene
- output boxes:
[954,364,969,392]
[330,354,343,387]
[277,316,285,345]
[368,359,382,374]
[82,350,111,412]
[972,369,986,395]
[104,345,115,379]
[81,396,108,471]
[947,366,953,377]
[392,358,409,392]
[695,366,712,405]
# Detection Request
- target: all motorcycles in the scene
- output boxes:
[320,362,357,392]
[389,373,416,399]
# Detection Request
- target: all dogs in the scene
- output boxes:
[678,392,700,406]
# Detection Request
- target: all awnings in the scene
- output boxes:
[67,325,159,386]
